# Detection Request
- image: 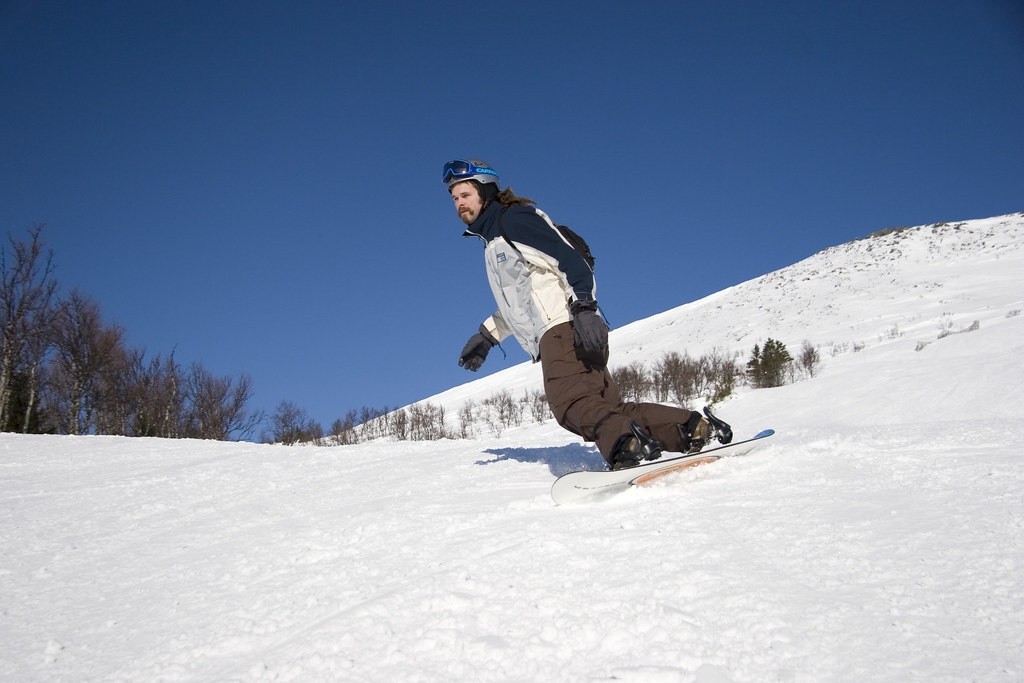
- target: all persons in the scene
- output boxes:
[442,158,733,472]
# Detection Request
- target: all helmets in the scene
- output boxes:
[448,160,501,193]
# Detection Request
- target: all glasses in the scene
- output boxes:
[441,159,499,183]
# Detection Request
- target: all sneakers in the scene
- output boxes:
[610,437,640,471]
[685,416,713,455]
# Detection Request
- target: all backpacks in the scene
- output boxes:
[497,204,595,271]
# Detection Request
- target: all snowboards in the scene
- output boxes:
[551,428,777,507]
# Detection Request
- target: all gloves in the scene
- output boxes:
[570,300,608,353]
[459,332,495,372]
